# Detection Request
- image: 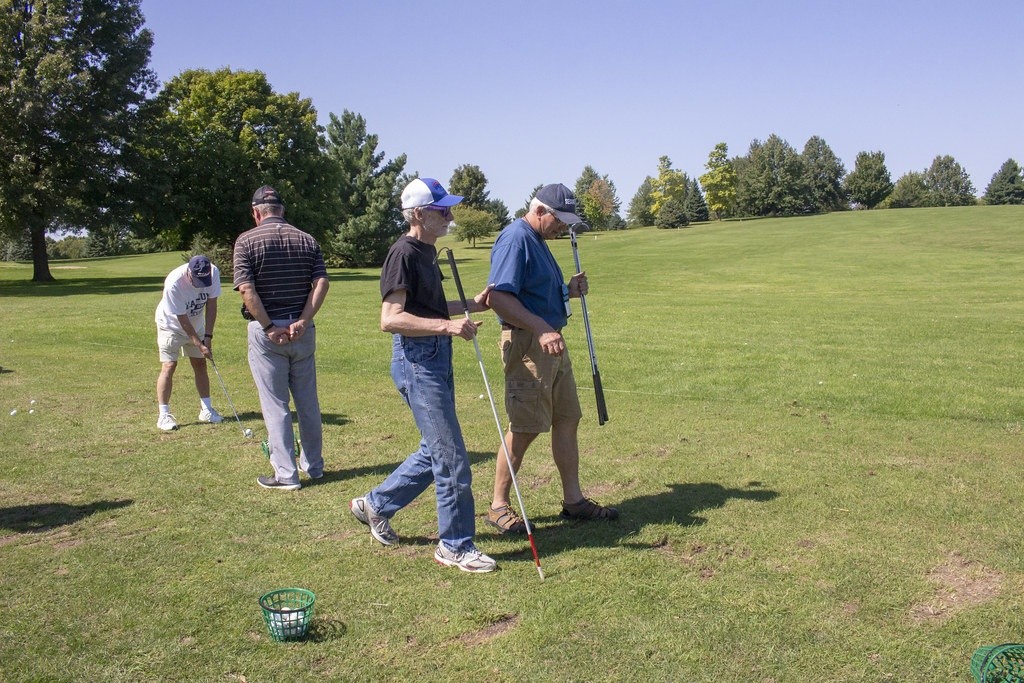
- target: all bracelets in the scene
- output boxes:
[262,322,274,332]
[204,334,213,339]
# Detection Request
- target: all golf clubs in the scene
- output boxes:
[208,357,256,441]
[567,220,609,426]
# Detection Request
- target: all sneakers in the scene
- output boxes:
[256,475,302,491]
[347,497,399,545]
[199,407,224,423]
[434,538,497,573]
[299,463,323,478]
[157,414,178,430]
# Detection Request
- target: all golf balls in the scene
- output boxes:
[245,428,252,436]
[10,399,37,416]
[269,606,308,642]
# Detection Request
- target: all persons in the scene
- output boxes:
[350,178,497,574]
[484,183,618,533]
[232,186,329,490]
[155,255,222,431]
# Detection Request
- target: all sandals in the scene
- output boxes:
[484,503,537,533]
[559,497,620,520]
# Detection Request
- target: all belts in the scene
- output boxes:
[252,312,303,321]
[500,322,525,331]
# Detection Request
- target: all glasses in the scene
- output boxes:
[420,202,452,216]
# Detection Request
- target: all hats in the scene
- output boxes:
[250,185,284,216]
[188,256,213,287]
[401,177,465,210]
[535,183,583,223]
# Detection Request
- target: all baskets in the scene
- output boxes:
[260,589,316,641]
